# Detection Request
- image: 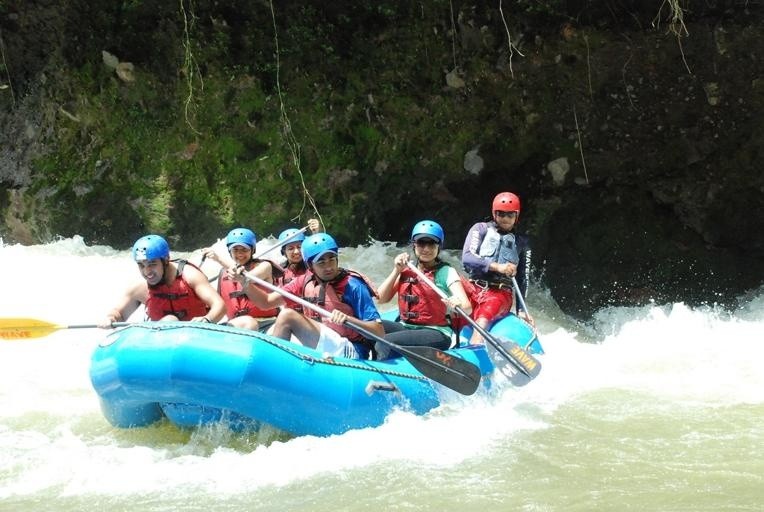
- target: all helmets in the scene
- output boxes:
[133,235,170,261]
[226,228,256,255]
[492,192,521,223]
[278,229,305,255]
[301,233,339,264]
[411,220,444,248]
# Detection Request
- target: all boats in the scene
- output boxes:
[88,308,545,440]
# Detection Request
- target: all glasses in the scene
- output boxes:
[497,211,515,218]
[416,240,436,247]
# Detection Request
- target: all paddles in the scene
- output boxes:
[242,272,480,395]
[0,318,133,339]
[405,265,540,385]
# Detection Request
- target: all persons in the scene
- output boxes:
[375,220,472,361]
[275,219,323,287]
[462,193,535,345]
[97,235,259,333]
[228,234,385,359]
[204,229,285,334]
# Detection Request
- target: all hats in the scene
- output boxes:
[308,248,338,263]
[414,233,439,243]
[229,242,252,250]
